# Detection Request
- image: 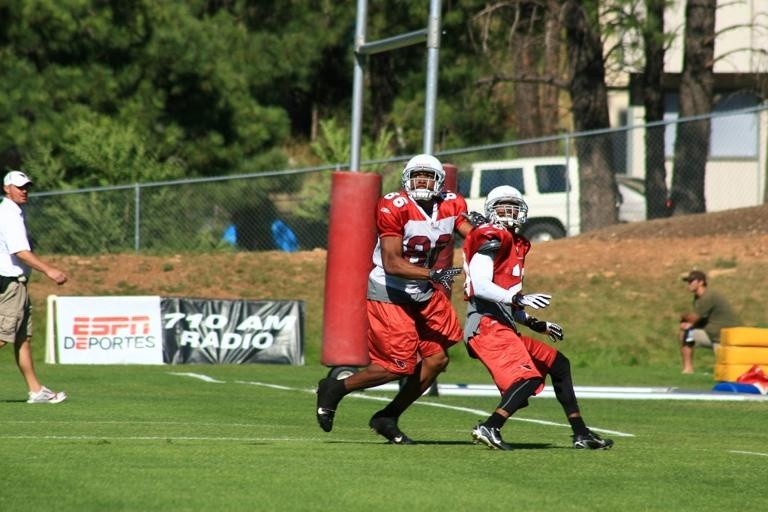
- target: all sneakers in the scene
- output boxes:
[27,386,67,404]
[573,428,614,450]
[369,410,414,445]
[316,378,342,432]
[470,423,514,450]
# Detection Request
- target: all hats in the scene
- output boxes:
[4,170,34,189]
[682,271,707,283]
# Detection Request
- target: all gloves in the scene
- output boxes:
[513,291,552,309]
[429,268,461,291]
[527,317,564,343]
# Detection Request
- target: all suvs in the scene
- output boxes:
[455,156,575,244]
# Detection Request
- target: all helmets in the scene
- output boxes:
[402,154,446,202]
[485,185,529,234]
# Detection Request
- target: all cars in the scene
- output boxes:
[609,175,672,225]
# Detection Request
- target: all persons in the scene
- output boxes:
[676,271,743,374]
[316,153,474,445]
[0,171,68,405]
[462,185,615,452]
